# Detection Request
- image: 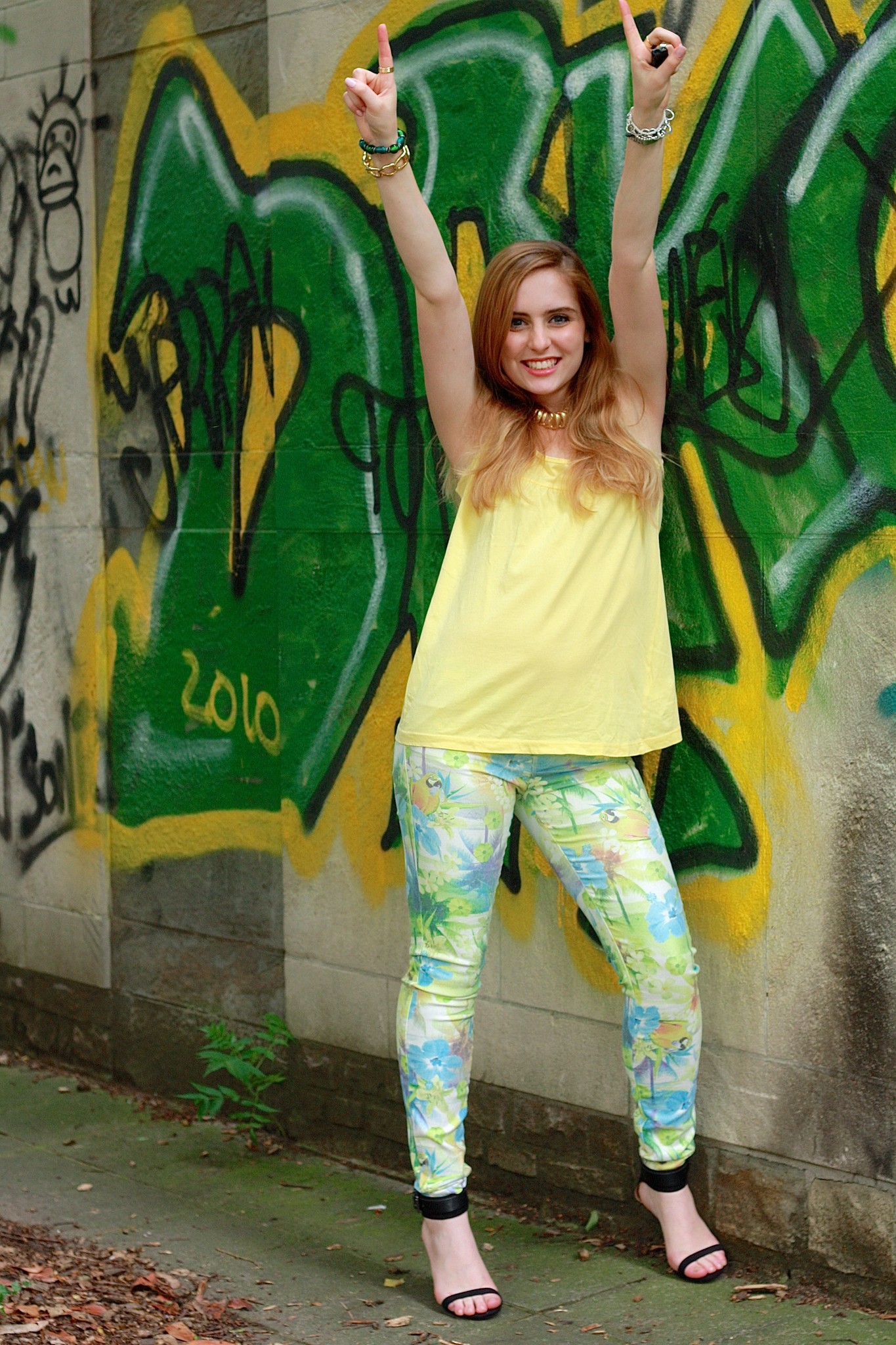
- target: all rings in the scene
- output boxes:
[645,36,653,46]
[378,66,394,73]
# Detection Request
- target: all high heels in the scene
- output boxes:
[415,1189,501,1319]
[634,1159,727,1283]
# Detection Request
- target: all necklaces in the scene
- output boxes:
[532,403,571,430]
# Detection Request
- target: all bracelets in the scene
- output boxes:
[626,107,674,151]
[363,145,410,178]
[359,128,404,155]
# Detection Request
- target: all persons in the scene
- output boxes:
[336,1,727,1318]
